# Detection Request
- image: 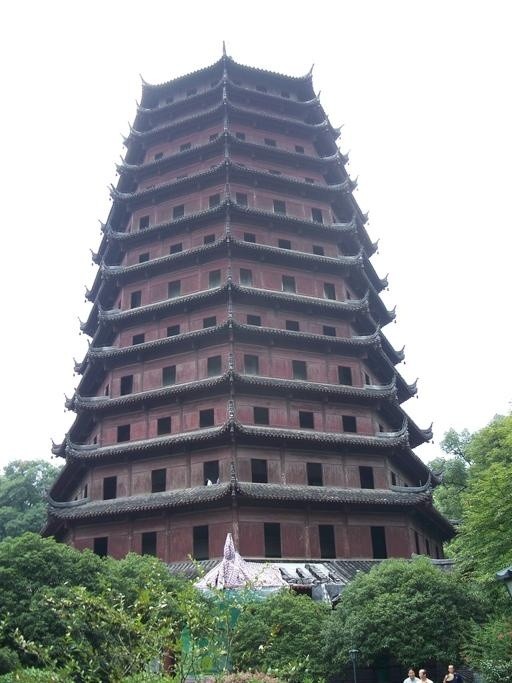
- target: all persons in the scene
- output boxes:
[416,669,433,683]
[216,478,220,484]
[443,665,465,683]
[403,668,419,683]
[206,478,212,485]
